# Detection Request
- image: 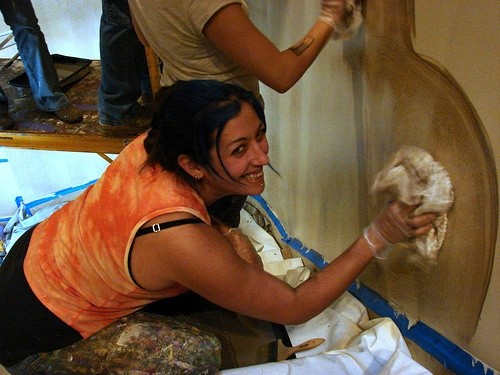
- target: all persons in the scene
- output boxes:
[0,0,82,133]
[96,0,159,136]
[128,0,347,229]
[0,76,441,367]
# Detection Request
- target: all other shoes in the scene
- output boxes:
[98,106,154,137]
[1,108,15,129]
[54,102,82,123]
